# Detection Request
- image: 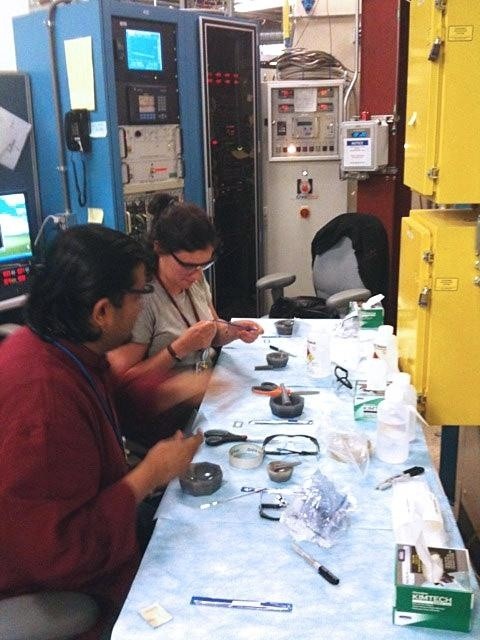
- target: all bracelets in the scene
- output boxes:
[167,344,185,362]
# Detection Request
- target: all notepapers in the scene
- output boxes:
[88,120,108,138]
[86,207,104,225]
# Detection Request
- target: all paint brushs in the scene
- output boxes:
[211,318,257,332]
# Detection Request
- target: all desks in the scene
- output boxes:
[107,317,479,636]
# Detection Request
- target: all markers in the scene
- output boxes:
[376,466,425,490]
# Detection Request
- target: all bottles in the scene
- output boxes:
[378,386,410,463]
[374,325,398,381]
[307,325,330,377]
[392,375,417,444]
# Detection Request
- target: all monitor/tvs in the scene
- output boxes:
[124,29,164,73]
[0,191,36,264]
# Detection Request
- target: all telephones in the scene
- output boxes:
[63,109,90,153]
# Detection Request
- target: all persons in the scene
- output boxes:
[107,194,263,404]
[0,225,205,640]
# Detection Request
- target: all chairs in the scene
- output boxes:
[255,212,389,320]
[0,591,101,639]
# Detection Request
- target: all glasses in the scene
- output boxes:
[129,283,154,294]
[170,251,216,272]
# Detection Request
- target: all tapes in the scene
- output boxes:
[227,442,266,470]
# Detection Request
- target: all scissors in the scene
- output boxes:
[251,381,294,397]
[204,428,266,447]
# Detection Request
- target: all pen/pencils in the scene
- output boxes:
[262,336,293,339]
[190,595,292,613]
[270,344,300,359]
[289,540,341,586]
